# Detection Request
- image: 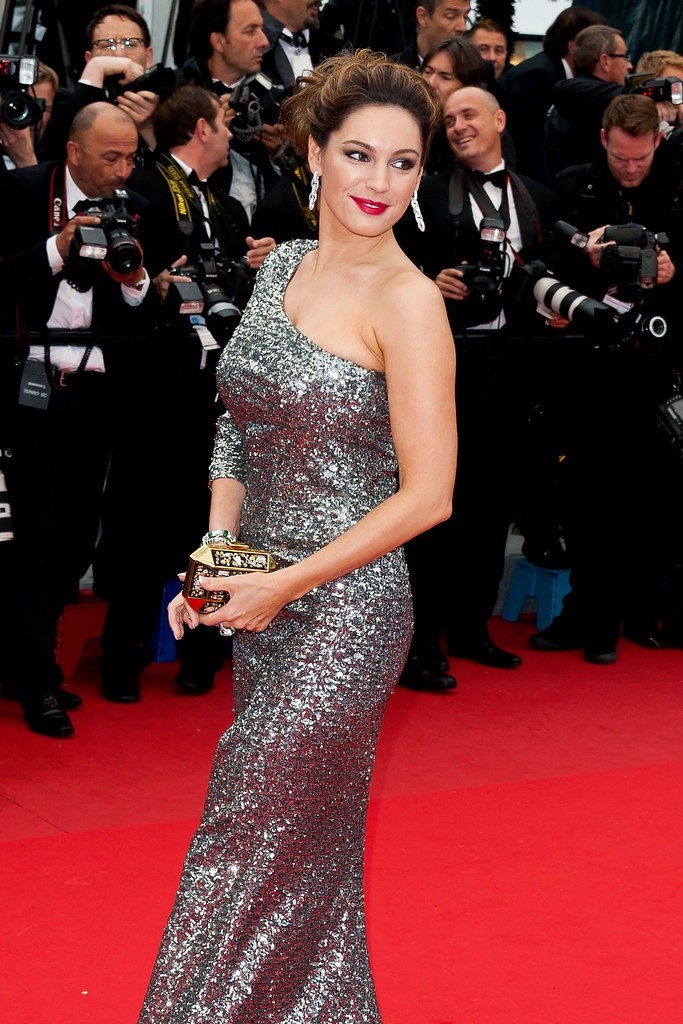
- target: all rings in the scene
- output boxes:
[218,622,236,637]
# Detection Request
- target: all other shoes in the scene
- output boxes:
[520,538,571,570]
[624,621,668,648]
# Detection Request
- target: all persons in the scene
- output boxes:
[398,90,547,692]
[0,100,151,739]
[107,87,275,703]
[137,53,457,1024]
[1,0,683,663]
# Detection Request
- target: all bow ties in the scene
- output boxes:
[212,81,233,96]
[473,169,507,190]
[188,169,208,196]
[279,31,309,48]
[72,198,118,215]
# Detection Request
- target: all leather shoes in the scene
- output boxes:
[447,629,523,669]
[177,657,213,694]
[22,692,73,737]
[411,634,449,670]
[51,688,82,708]
[529,614,590,650]
[585,627,617,664]
[401,661,456,692]
[102,652,141,705]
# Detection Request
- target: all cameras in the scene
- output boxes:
[73,188,142,274]
[510,223,670,341]
[455,217,507,302]
[0,54,43,130]
[658,394,683,445]
[633,77,683,151]
[171,241,242,341]
[103,66,180,105]
[230,72,282,129]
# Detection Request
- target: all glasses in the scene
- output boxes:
[602,51,631,62]
[89,36,147,55]
